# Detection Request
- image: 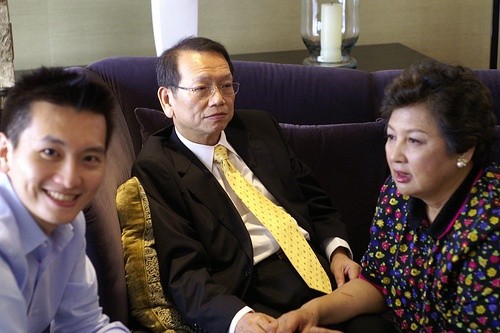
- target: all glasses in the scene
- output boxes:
[172,82,240,99]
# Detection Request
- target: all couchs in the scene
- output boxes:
[81,55,500,333]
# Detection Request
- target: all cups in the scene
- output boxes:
[300,0,361,68]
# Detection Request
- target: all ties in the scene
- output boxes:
[213,144,333,295]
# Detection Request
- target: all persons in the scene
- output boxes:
[0,67,133,333]
[272,61,500,333]
[146,37,362,333]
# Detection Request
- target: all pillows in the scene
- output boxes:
[133,107,389,240]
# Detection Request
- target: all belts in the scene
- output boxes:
[254,249,286,269]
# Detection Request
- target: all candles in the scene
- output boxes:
[317,3,345,63]
